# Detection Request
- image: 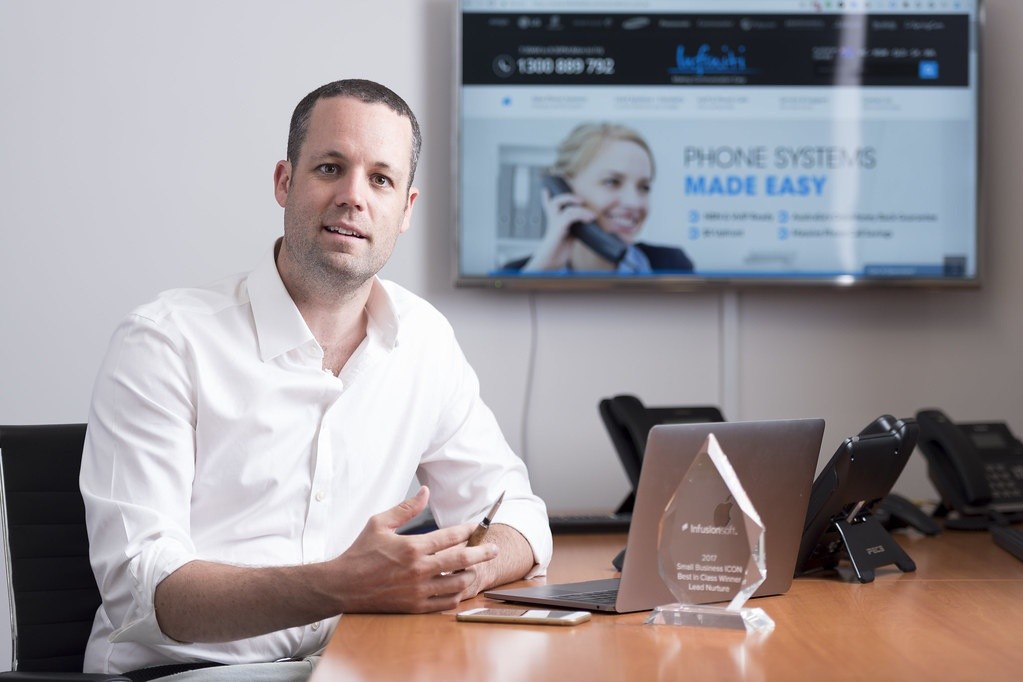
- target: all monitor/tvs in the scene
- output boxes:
[450,1,981,290]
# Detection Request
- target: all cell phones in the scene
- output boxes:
[457,607,592,626]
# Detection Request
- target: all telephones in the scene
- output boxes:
[598,392,727,517]
[914,409,1023,532]
[544,174,627,262]
[792,414,937,584]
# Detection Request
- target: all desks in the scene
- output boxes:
[307,525,1023,682]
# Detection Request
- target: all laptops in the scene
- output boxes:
[483,417,826,614]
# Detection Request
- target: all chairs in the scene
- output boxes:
[2,420,223,682]
[599,396,727,518]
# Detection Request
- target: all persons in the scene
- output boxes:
[78,80,554,675]
[502,123,694,271]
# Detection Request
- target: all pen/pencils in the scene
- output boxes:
[451,490,505,577]
